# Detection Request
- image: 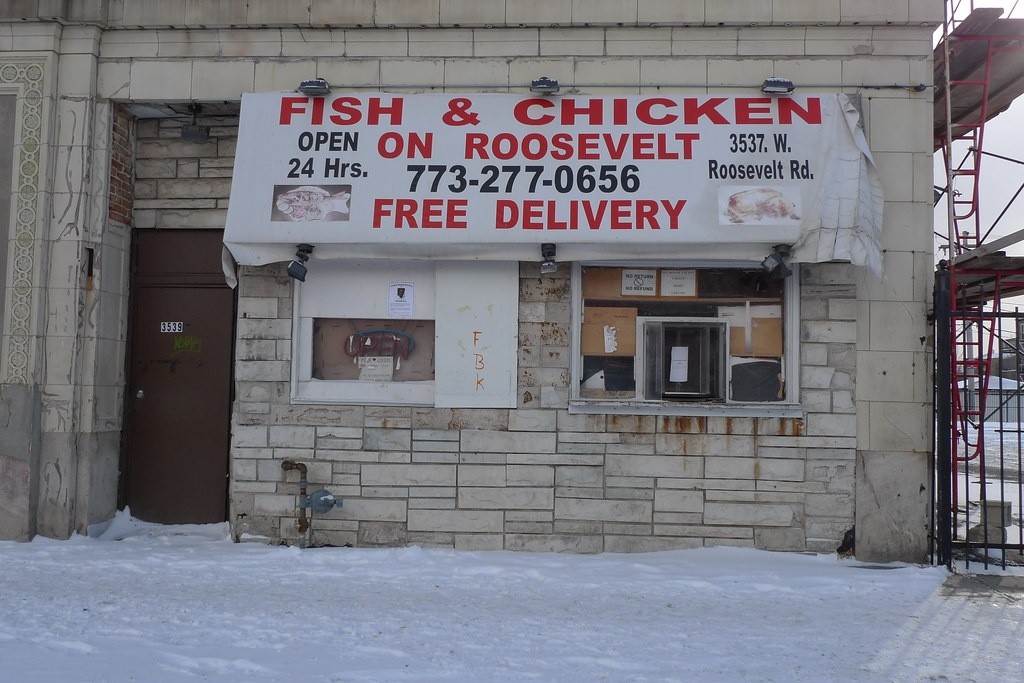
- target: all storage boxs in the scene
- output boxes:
[579,270,781,357]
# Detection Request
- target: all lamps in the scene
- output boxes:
[530,77,561,96]
[539,244,557,276]
[762,78,794,94]
[180,103,211,140]
[299,77,331,96]
[285,242,317,283]
[761,246,796,279]
